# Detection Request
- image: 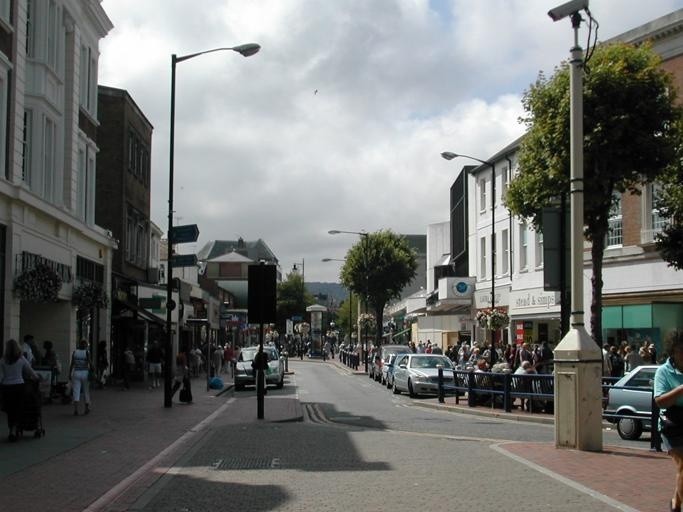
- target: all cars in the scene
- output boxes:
[602,364,667,441]
[231,347,286,390]
[368,344,461,399]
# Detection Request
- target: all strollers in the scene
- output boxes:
[3,372,46,439]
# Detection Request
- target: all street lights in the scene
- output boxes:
[440,151,497,368]
[292,258,305,314]
[321,258,353,353]
[327,228,369,373]
[163,41,264,409]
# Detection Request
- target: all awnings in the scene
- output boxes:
[393,328,410,337]
[382,332,397,337]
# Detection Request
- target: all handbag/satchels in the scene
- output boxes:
[659,405,682,449]
[64,380,72,396]
[179,380,192,402]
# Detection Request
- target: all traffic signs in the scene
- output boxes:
[168,254,198,266]
[169,221,199,243]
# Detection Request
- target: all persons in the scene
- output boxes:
[94,339,109,387]
[651,327,682,512]
[117,337,248,403]
[264,327,310,357]
[408,340,442,355]
[0,338,39,441]
[324,336,378,364]
[603,338,658,410]
[445,340,555,414]
[20,334,38,367]
[67,339,95,416]
[251,346,268,394]
[41,339,61,404]
